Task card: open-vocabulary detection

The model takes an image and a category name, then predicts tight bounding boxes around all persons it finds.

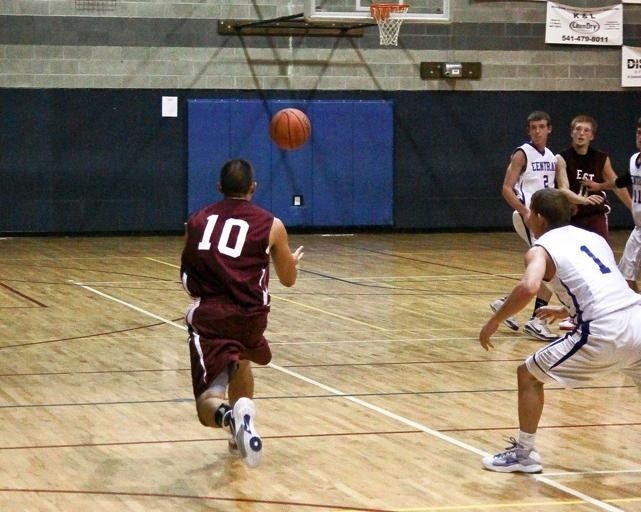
[479,188,641,474]
[487,110,560,341]
[555,115,631,329]
[178,160,305,469]
[619,120,641,291]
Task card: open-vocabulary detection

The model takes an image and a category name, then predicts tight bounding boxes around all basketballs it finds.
[269,107,310,151]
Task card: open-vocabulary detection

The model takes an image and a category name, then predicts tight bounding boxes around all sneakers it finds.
[228,435,243,457]
[488,294,522,332]
[558,314,577,330]
[231,396,264,466]
[481,438,544,474]
[522,317,562,343]
